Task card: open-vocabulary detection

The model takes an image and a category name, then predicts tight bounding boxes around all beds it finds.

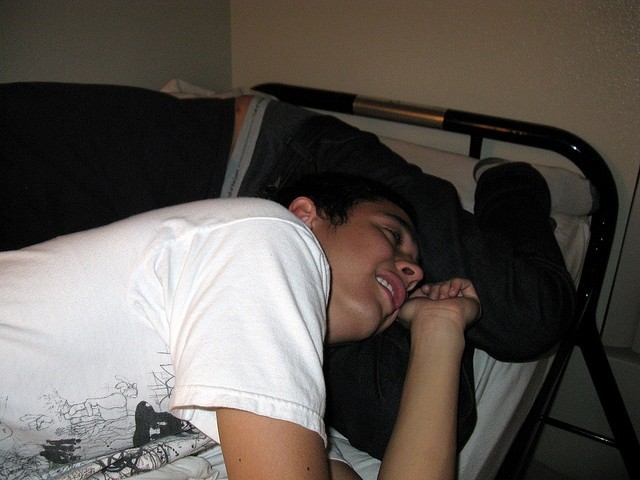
[0,81,640,478]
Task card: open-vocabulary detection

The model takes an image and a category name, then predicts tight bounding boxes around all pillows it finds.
[0,81,235,254]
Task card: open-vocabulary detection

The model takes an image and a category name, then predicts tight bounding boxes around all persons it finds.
[0,182,481,480]
